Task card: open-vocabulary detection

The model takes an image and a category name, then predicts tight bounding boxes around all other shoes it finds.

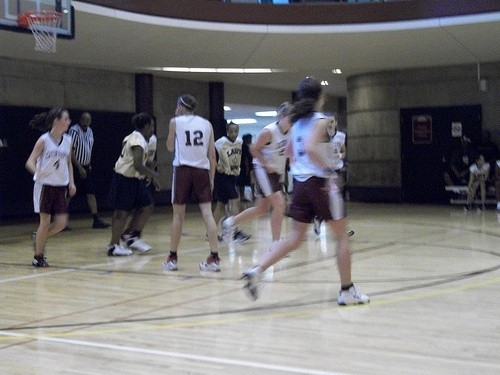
[93,222,110,229]
[61,226,72,232]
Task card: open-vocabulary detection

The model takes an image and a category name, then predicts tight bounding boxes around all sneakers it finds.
[219,216,240,247]
[161,256,177,270]
[199,255,220,271]
[338,287,370,305]
[31,232,37,251]
[120,233,152,252]
[32,254,49,267]
[347,231,354,237]
[314,216,321,234]
[241,272,259,300]
[106,243,132,256]
[234,231,252,244]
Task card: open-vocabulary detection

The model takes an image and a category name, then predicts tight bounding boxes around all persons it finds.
[465,154,490,212]
[494,160,500,210]
[25,106,76,267]
[218,100,294,256]
[107,112,158,256]
[313,112,356,239]
[240,134,257,203]
[242,77,368,306]
[206,121,250,243]
[281,157,290,192]
[164,94,221,272]
[61,111,109,231]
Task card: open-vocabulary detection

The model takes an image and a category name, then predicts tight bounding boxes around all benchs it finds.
[445,186,497,204]
